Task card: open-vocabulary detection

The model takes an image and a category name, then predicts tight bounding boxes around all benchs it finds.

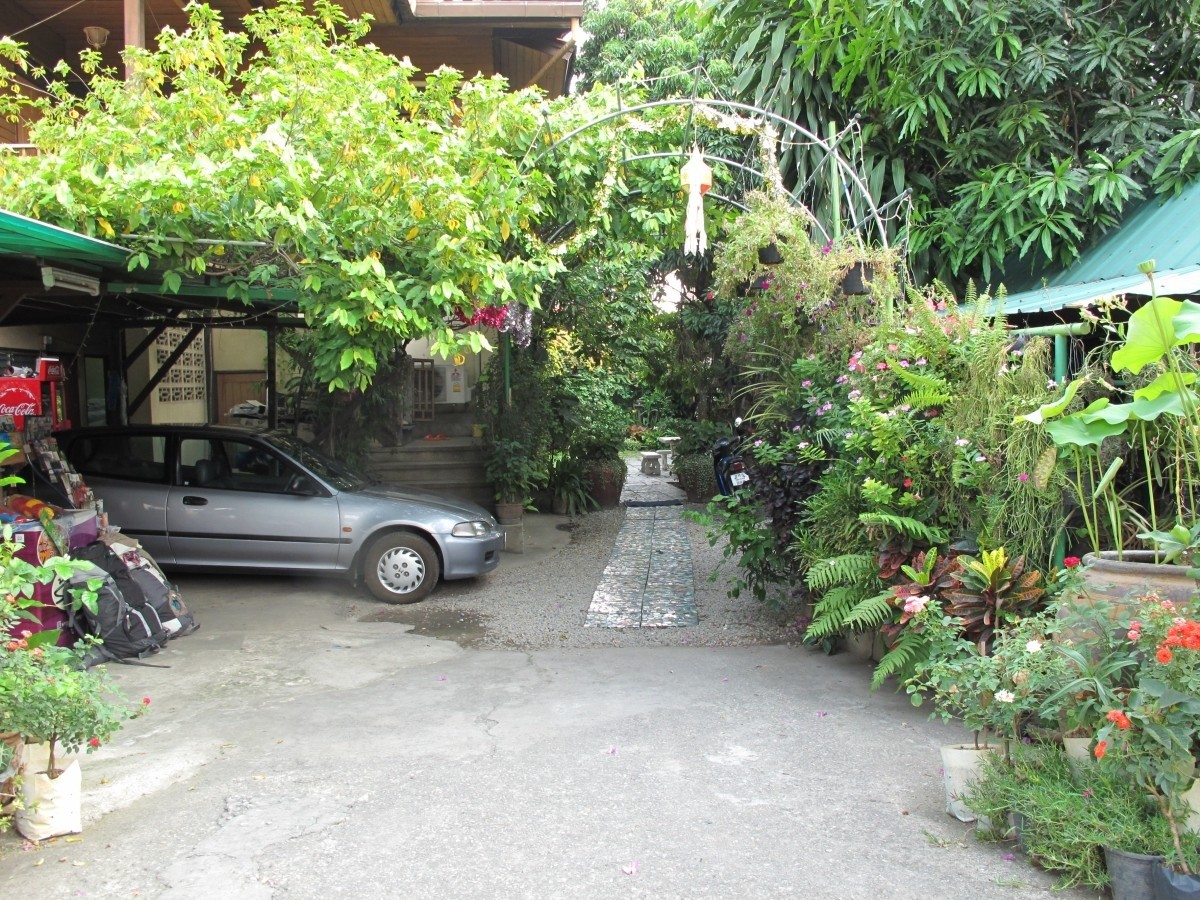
[657,450,671,470]
[641,451,662,476]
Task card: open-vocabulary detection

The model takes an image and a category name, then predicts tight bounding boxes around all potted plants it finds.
[485,439,539,523]
[548,459,604,516]
[0,441,102,802]
[901,255,1198,730]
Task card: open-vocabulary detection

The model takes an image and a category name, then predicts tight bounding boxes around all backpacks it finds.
[54,541,171,668]
[96,532,201,639]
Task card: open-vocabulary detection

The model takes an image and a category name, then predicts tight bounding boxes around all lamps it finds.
[41,266,100,297]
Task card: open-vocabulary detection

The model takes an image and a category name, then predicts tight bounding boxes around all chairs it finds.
[195,459,226,488]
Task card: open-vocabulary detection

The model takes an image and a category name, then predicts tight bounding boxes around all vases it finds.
[1152,857,1200,900]
[14,757,84,840]
[1098,842,1164,900]
[1062,732,1094,776]
[943,747,1004,822]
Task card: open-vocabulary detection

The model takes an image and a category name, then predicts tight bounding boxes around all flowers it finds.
[892,552,1198,884]
[0,639,151,779]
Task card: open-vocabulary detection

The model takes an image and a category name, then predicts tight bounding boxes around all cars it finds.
[2,424,507,605]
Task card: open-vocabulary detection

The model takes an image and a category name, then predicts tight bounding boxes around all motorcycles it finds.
[703,417,752,512]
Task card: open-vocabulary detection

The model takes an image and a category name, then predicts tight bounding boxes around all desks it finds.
[657,437,682,459]
